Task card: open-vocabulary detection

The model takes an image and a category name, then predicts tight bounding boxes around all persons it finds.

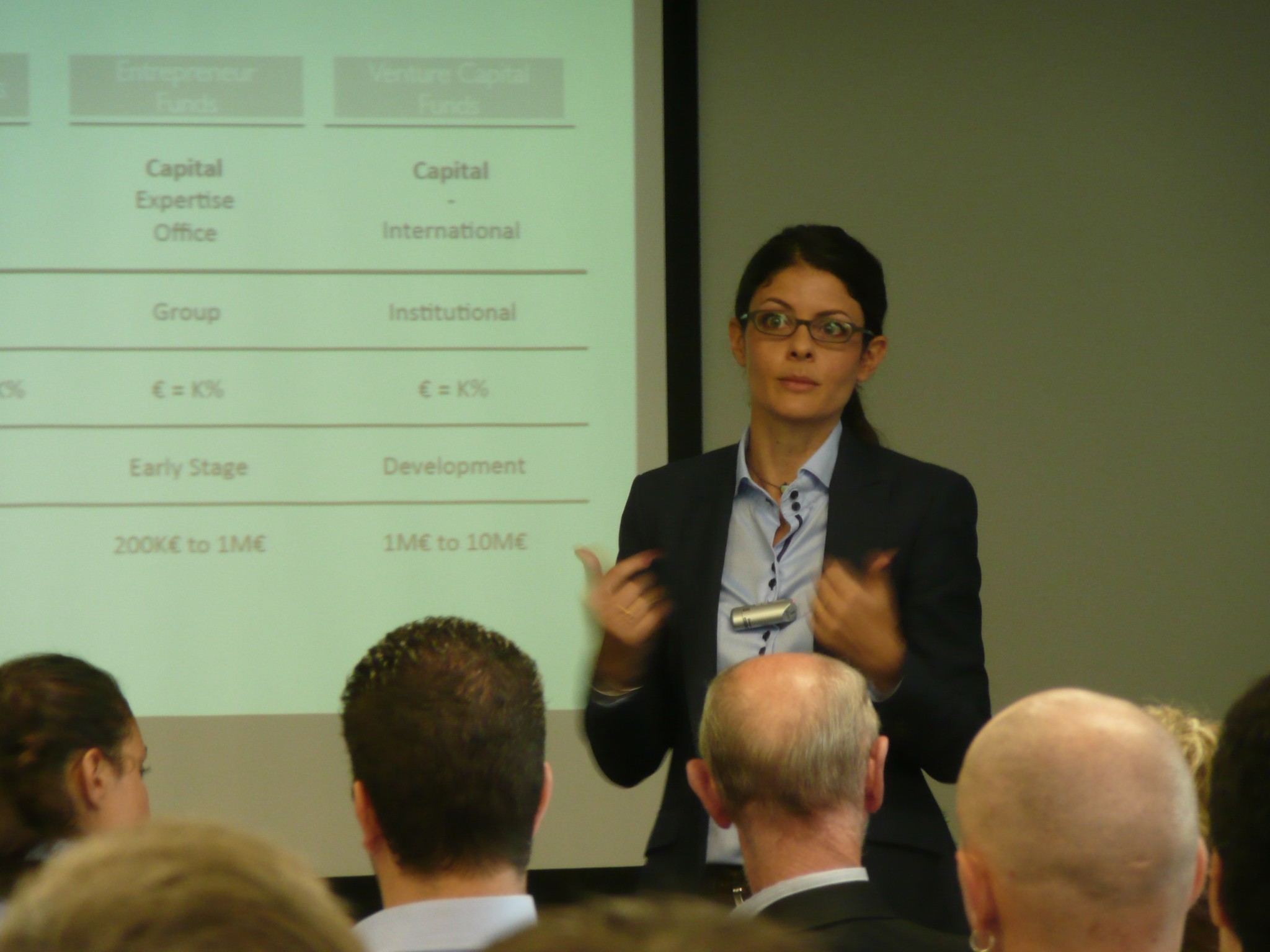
[0,615,1270,952]
[574,225,991,937]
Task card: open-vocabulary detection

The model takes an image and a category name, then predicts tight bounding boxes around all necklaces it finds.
[746,454,790,493]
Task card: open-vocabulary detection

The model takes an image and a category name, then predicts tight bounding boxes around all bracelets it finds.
[588,677,642,698]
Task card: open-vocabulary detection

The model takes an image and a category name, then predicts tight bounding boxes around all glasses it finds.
[738,309,872,342]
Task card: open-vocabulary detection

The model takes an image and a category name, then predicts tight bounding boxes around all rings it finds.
[623,607,641,620]
